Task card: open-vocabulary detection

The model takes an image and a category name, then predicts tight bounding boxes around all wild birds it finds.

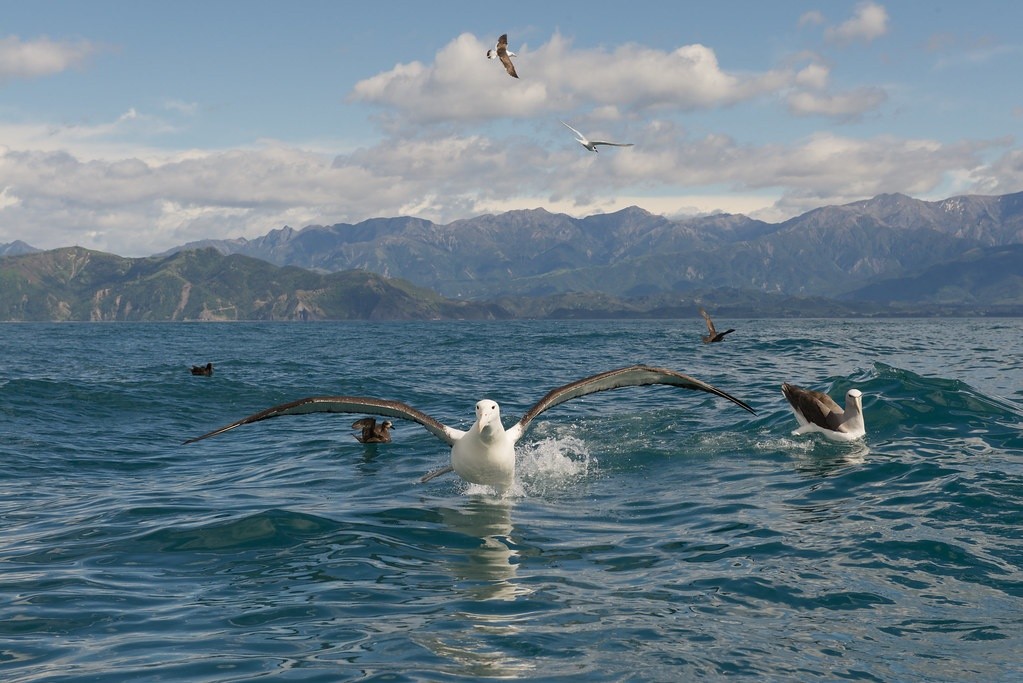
[487,33,519,79]
[351,416,396,445]
[180,362,758,498]
[701,309,735,343]
[559,120,634,155]
[781,380,867,443]
[189,363,214,377]
